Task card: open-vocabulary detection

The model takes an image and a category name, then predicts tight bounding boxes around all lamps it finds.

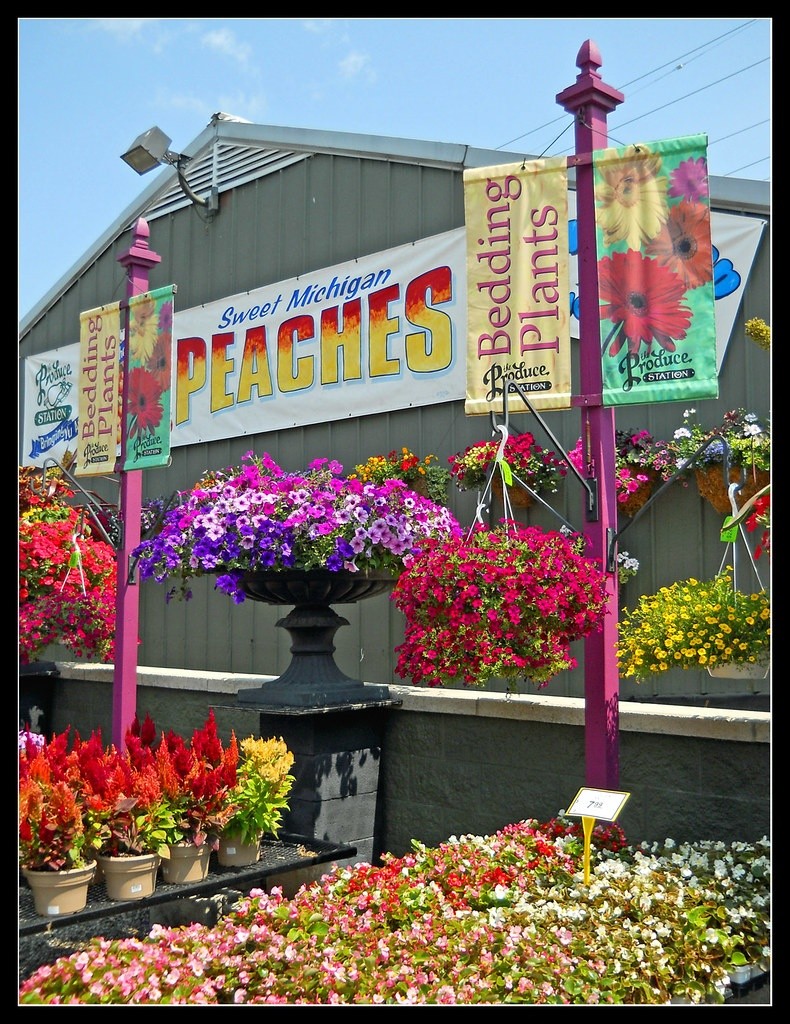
[120,125,173,176]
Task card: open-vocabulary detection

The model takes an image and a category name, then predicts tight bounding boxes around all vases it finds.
[217,828,264,867]
[695,464,770,515]
[400,476,429,500]
[489,468,535,508]
[160,840,213,885]
[22,859,97,917]
[707,652,770,681]
[97,852,162,902]
[727,963,770,998]
[616,463,663,518]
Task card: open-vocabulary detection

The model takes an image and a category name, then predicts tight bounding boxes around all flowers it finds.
[643,200,714,290]
[129,292,158,365]
[132,449,460,603]
[158,300,172,333]
[19,706,770,1005]
[146,331,171,392]
[560,525,640,585]
[594,144,670,252]
[448,433,568,494]
[613,566,770,684]
[653,408,770,488]
[745,496,770,561]
[346,446,454,505]
[19,488,142,664]
[127,366,164,439]
[667,156,710,202]
[564,428,656,502]
[389,516,613,690]
[598,248,694,358]
